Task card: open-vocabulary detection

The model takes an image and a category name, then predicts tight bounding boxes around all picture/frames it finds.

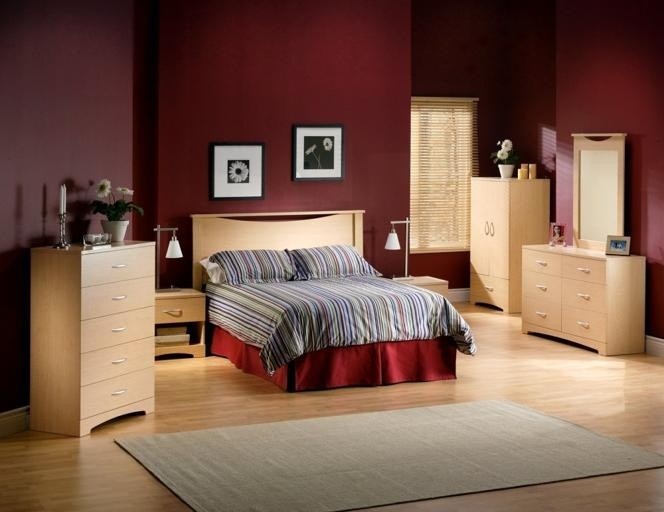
[291,121,347,183]
[604,235,632,257]
[206,139,266,202]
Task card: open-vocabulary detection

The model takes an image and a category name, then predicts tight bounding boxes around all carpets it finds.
[112,398,663,511]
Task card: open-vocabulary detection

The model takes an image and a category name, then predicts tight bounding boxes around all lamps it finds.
[384,216,415,280]
[154,222,185,293]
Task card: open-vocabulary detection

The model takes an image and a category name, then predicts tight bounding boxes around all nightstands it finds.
[399,276,449,298]
[155,287,207,359]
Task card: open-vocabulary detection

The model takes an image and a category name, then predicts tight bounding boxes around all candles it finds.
[64,185,66,213]
[60,185,64,216]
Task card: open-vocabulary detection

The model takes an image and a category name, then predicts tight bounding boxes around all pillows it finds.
[208,247,296,284]
[290,244,384,280]
[200,254,226,284]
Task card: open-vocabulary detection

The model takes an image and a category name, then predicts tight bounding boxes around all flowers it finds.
[90,178,145,219]
[303,137,333,169]
[226,160,249,184]
[495,139,513,163]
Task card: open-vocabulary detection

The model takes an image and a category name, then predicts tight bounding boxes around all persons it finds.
[553,226,561,238]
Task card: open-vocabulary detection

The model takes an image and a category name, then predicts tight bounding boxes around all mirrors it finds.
[570,131,627,252]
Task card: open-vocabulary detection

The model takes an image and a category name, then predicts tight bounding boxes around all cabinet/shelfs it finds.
[469,175,552,315]
[28,238,158,438]
[519,242,647,357]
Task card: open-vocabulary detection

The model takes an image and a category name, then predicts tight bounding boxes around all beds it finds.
[187,209,478,394]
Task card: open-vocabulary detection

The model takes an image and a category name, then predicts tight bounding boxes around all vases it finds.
[497,164,515,177]
[100,218,131,243]
